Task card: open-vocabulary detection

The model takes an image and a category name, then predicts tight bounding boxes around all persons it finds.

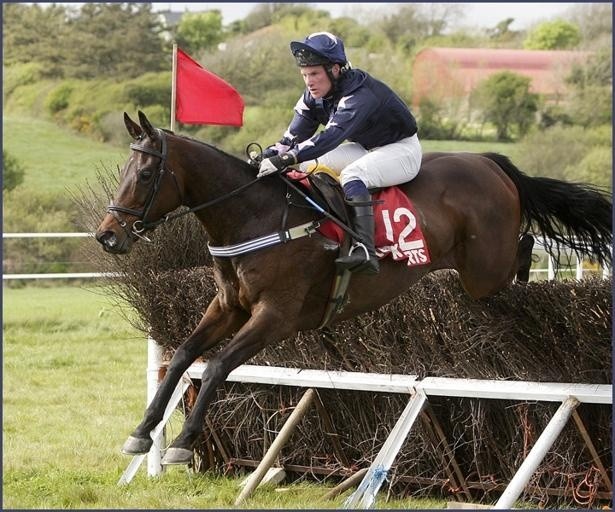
[247,32,424,277]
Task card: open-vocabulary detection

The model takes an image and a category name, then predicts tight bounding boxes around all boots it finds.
[331,193,379,276]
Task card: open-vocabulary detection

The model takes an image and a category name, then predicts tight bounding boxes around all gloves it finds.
[247,148,279,169]
[254,150,299,180]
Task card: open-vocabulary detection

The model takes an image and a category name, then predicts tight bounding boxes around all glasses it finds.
[294,49,334,67]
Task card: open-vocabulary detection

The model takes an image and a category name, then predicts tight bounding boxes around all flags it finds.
[174,44,245,128]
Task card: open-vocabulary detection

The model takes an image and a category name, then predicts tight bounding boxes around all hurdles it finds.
[70,156,614,510]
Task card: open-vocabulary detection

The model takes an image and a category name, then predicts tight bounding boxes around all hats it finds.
[288,31,347,64]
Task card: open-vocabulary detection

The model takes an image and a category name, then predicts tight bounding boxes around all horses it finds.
[94,108,614,467]
